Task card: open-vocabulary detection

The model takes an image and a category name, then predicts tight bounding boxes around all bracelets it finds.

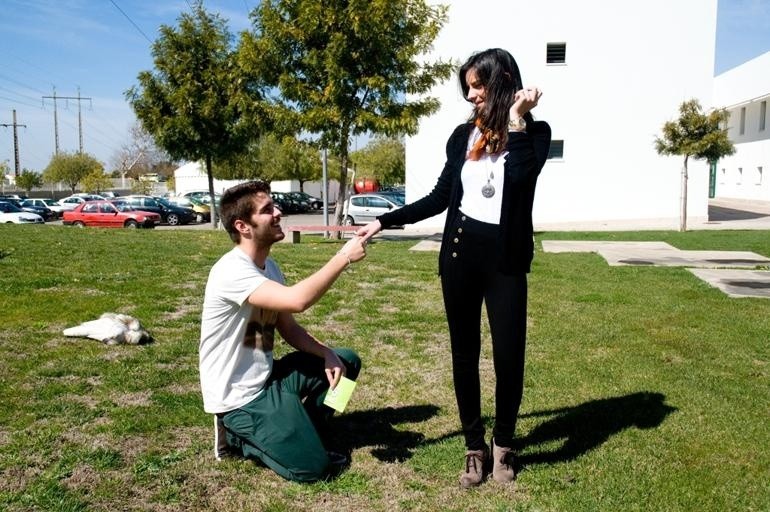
[336,250,351,270]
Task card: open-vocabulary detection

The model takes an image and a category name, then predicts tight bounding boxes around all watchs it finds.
[507,117,527,131]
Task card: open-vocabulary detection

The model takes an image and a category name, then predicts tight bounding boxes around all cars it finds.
[335,188,405,226]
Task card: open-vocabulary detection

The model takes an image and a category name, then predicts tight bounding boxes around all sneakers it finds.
[492,436,515,484]
[461,445,489,489]
[214,414,233,463]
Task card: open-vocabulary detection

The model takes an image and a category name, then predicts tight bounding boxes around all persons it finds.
[355,47,551,487]
[198,180,366,484]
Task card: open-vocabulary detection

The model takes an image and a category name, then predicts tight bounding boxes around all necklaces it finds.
[482,153,500,197]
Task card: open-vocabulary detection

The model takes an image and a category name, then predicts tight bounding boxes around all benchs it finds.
[286,225,373,244]
